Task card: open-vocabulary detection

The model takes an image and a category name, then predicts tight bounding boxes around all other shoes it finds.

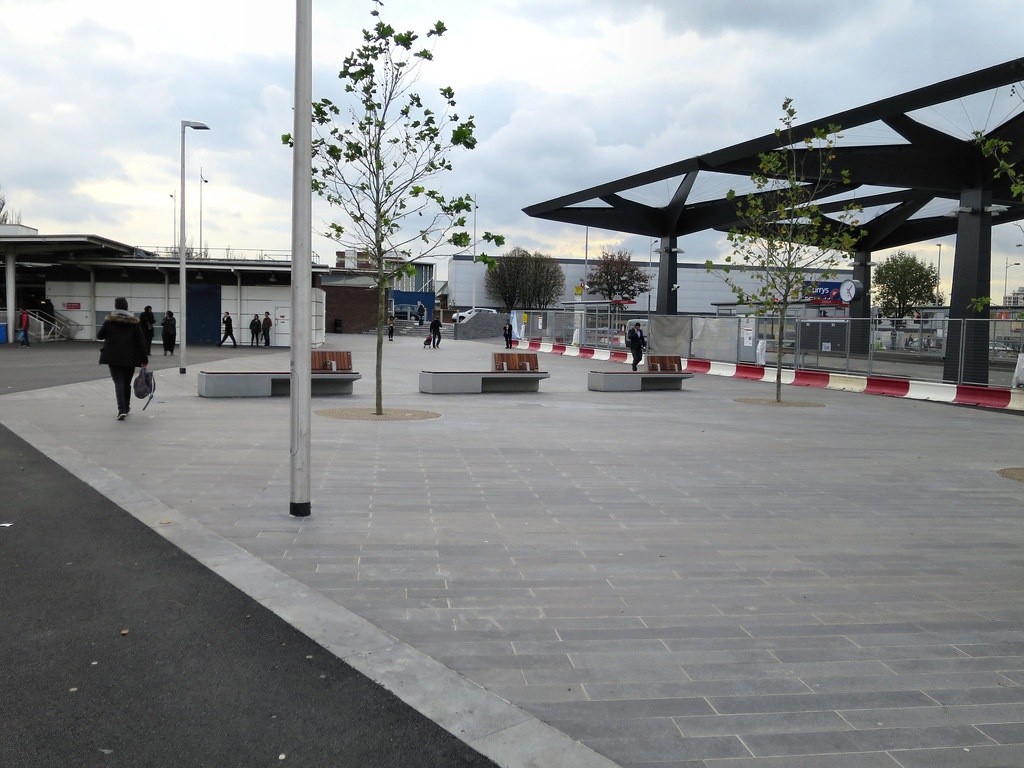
[231,346,237,348]
[216,342,220,347]
[164,353,173,355]
[389,338,393,341]
[433,345,439,348]
[263,346,270,349]
[20,345,30,349]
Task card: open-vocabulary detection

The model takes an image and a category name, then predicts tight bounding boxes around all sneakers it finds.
[117,407,130,420]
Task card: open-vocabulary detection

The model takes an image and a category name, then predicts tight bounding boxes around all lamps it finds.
[120,268,129,277]
[653,248,664,253]
[672,247,686,255]
[944,212,959,218]
[195,272,203,280]
[848,262,861,267]
[868,262,879,267]
[951,207,973,214]
[987,204,1012,216]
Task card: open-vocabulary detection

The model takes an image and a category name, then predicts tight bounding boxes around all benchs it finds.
[588,355,695,392]
[197,351,361,399]
[419,352,550,394]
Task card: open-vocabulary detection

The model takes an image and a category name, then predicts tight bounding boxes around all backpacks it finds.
[17,330,24,341]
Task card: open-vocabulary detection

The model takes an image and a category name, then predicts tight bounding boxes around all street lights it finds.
[179,120,210,373]
[1003,263,1020,306]
[472,205,479,309]
[937,243,942,306]
[648,240,659,311]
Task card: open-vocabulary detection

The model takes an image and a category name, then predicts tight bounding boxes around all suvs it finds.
[386,304,424,321]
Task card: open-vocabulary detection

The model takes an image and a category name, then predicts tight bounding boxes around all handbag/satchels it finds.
[133,365,155,399]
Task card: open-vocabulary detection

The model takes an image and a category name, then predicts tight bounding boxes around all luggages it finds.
[424,333,432,348]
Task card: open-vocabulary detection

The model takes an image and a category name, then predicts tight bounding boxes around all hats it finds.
[266,312,271,315]
[115,297,127,309]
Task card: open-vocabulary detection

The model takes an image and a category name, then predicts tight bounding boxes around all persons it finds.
[387,316,395,341]
[140,305,156,355]
[503,319,512,349]
[18,298,55,348]
[262,311,272,347]
[873,334,930,351]
[216,311,237,348]
[97,297,149,422]
[430,316,442,349]
[161,310,177,356]
[627,323,646,371]
[249,314,262,347]
[418,305,425,327]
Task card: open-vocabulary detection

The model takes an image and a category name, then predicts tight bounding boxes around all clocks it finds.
[840,280,855,301]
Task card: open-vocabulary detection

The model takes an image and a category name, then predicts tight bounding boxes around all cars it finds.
[452,308,497,323]
[989,342,1013,353]
